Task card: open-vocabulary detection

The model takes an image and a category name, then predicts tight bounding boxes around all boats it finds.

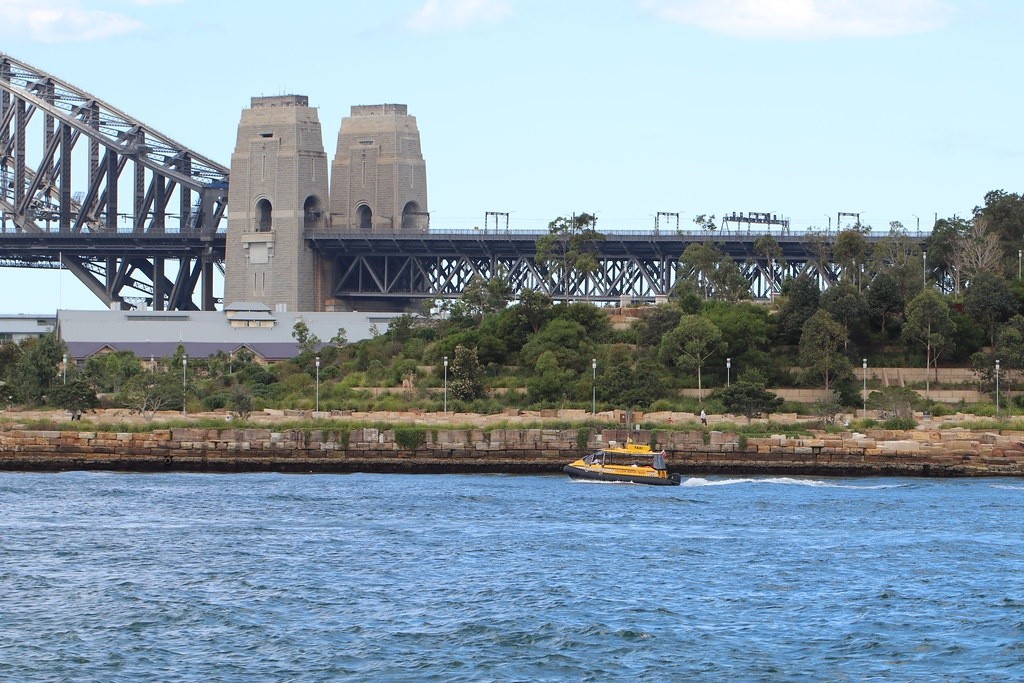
[563,437,681,487]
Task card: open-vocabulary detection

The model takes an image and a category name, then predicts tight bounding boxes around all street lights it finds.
[444,356,448,414]
[151,354,154,374]
[863,359,868,420]
[183,356,187,414]
[727,358,730,387]
[592,359,596,415]
[316,357,319,413]
[996,360,1000,413]
[63,354,67,385]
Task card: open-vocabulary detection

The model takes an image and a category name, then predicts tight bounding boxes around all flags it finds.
[662,451,666,458]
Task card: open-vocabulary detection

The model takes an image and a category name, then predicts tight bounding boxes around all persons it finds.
[700,408,706,425]
[593,457,600,463]
[630,462,637,467]
